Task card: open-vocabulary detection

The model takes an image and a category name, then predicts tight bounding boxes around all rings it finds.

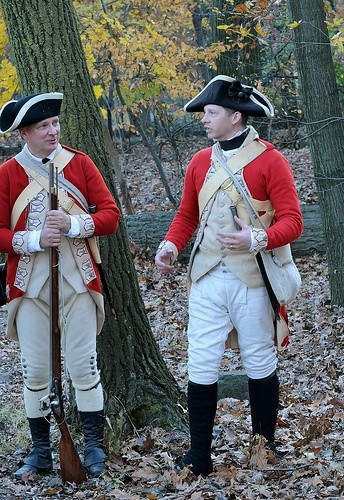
[231,245,233,248]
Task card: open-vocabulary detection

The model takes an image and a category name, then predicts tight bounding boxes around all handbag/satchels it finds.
[254,243,302,306]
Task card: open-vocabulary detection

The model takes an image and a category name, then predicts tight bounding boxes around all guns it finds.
[39,161,88,483]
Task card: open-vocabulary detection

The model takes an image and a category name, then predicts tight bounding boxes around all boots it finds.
[173,382,218,476]
[14,413,53,480]
[79,409,109,477]
[247,372,280,448]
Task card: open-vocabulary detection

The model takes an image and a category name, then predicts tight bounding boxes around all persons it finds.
[154,74,304,478]
[0,91,120,480]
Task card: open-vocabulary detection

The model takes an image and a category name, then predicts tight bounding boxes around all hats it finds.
[0,93,65,134]
[184,75,275,117]
